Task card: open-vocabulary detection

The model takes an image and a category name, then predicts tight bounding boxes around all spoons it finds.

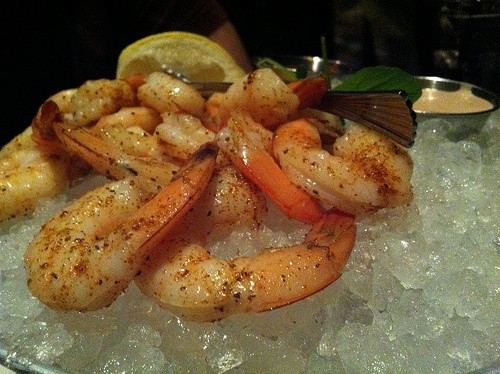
[159,67,418,150]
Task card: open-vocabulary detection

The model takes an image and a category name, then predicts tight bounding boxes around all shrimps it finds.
[2,69,415,325]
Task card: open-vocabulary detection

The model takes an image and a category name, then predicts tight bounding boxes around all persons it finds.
[0,0,252,151]
[336,0,405,69]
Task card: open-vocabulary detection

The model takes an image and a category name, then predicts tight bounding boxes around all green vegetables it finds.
[329,64,423,108]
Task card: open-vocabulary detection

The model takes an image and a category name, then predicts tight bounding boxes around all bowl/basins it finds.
[411,75,500,138]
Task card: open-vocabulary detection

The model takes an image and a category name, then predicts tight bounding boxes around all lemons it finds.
[116,30,244,87]
